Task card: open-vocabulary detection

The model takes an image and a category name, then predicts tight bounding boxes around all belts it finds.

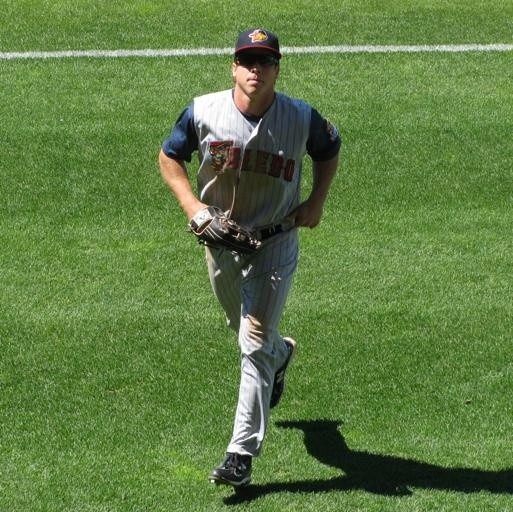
[252,220,293,240]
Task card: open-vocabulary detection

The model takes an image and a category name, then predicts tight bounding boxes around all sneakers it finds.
[269,337,297,409]
[207,452,252,486]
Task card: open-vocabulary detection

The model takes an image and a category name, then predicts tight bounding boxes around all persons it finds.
[158,28,341,486]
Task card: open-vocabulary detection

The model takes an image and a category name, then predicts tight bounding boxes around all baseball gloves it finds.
[189,205,262,255]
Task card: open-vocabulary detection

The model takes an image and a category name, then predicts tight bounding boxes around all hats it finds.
[235,28,281,59]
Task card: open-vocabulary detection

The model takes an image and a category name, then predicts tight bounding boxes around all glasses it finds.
[235,53,278,66]
